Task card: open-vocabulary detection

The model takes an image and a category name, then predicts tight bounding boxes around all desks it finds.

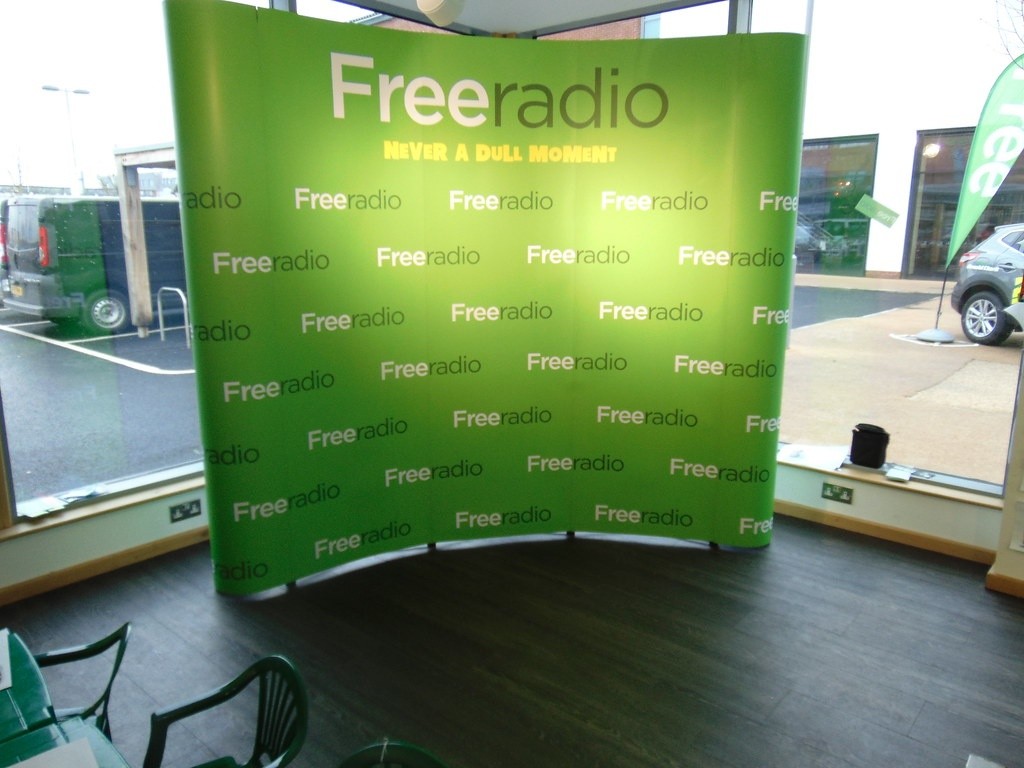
[0,632,132,768]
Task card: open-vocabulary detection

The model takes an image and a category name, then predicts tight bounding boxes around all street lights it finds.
[41,83,92,190]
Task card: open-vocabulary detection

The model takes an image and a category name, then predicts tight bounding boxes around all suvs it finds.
[948,222,1024,347]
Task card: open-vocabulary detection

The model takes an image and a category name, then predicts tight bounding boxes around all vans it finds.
[0,189,187,338]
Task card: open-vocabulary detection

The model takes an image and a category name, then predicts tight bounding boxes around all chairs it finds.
[336,740,450,768]
[34,620,133,743]
[142,654,309,768]
